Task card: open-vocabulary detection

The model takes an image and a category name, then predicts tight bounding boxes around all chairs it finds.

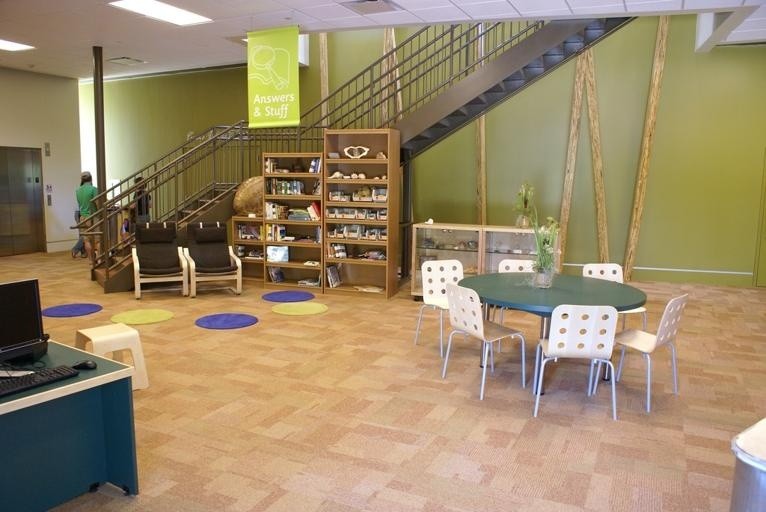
[582,263,647,333]
[183,221,243,298]
[605,293,688,414]
[532,304,619,420]
[442,283,526,400]
[492,259,536,353]
[132,222,188,299]
[415,259,464,357]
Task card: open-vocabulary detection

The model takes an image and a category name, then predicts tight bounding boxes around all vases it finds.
[515,215,530,229]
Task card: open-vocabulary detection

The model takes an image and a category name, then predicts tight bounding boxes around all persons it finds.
[125,176,152,235]
[76,171,108,266]
[71,181,89,261]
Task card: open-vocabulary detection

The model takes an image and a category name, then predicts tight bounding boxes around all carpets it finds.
[195,313,258,329]
[262,291,315,302]
[41,304,103,317]
[110,309,174,325]
[272,302,328,316]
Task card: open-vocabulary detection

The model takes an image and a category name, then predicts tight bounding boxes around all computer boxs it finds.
[0,342,48,366]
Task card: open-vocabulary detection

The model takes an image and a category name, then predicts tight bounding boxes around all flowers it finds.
[511,180,536,217]
[528,216,558,273]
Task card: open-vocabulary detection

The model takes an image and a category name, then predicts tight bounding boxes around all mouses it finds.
[73,359,97,370]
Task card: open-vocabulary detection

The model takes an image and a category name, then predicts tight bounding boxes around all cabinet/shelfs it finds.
[482,226,561,278]
[262,152,322,293]
[411,222,481,300]
[231,216,264,284]
[324,127,400,300]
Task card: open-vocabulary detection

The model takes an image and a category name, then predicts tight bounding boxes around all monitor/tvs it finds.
[0,279,48,353]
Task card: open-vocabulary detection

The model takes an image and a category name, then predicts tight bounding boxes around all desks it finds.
[454,272,646,396]
[0,341,141,512]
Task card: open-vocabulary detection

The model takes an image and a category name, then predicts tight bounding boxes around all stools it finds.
[76,322,149,392]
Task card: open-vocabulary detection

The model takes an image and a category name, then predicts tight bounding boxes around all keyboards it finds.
[0,365,79,396]
